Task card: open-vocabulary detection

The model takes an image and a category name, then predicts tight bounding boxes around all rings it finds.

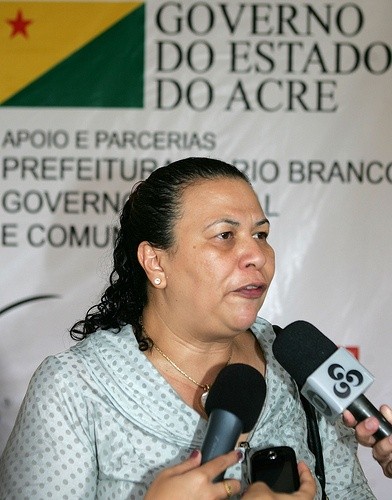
[224,480,233,497]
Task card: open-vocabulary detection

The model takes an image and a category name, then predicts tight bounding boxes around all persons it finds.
[0,156,377,500]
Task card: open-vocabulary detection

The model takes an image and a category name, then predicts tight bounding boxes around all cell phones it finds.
[250,445,300,494]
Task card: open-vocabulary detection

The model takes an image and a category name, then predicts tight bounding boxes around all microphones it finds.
[272,320,392,441]
[198,364,266,484]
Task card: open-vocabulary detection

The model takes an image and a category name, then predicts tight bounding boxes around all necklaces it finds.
[139,315,234,409]
